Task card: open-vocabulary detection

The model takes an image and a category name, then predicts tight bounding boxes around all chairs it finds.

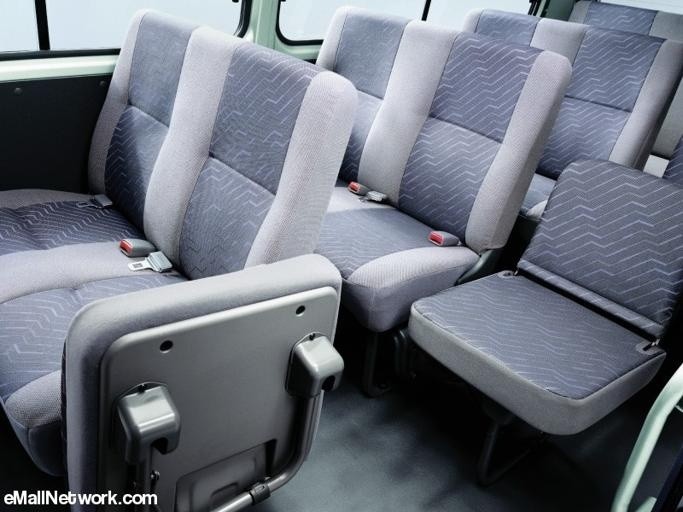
[0,0,682,511]
